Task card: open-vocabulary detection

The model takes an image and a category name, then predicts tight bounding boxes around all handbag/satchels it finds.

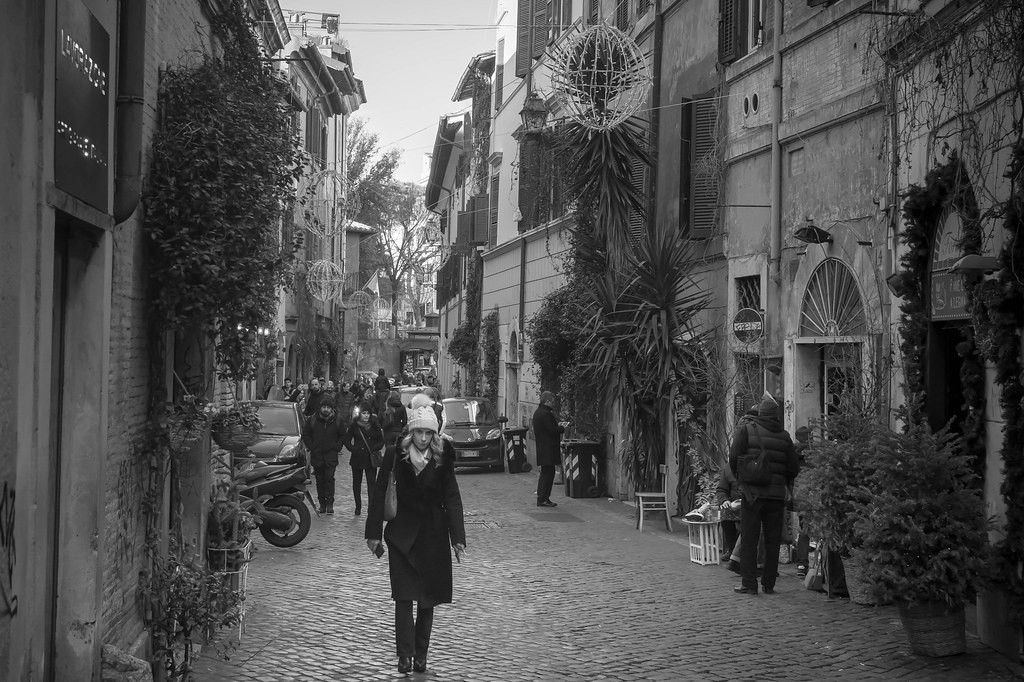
[370,451,383,467]
[384,451,398,520]
[737,423,768,484]
[381,402,394,428]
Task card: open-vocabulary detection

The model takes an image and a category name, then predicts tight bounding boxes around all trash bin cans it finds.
[565,440,603,498]
[560,439,588,496]
[502,425,532,474]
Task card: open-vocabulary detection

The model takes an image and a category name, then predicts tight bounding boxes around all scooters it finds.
[231,461,320,549]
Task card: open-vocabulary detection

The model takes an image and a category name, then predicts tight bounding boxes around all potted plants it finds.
[212,401,263,452]
[791,393,1005,657]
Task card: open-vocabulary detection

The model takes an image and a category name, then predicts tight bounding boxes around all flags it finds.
[368,275,379,293]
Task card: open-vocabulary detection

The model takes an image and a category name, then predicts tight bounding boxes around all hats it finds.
[409,394,438,433]
[360,401,373,414]
[760,391,779,415]
[320,394,333,410]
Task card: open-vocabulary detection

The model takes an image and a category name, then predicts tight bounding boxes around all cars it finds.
[439,397,509,474]
[395,387,442,413]
[234,399,314,476]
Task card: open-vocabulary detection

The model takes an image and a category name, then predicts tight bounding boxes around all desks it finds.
[682,518,724,565]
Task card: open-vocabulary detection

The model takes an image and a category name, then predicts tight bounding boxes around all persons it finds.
[365,394,466,672]
[718,397,814,595]
[265,368,437,514]
[533,391,569,507]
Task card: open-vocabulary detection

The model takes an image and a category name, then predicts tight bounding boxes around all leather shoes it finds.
[761,585,774,593]
[413,657,426,672]
[734,585,758,594]
[398,657,411,672]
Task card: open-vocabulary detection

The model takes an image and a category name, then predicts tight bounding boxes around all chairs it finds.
[634,464,673,533]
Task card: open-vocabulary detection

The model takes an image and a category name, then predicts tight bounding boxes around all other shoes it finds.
[355,507,361,515]
[797,565,807,575]
[537,499,557,507]
[319,498,326,513]
[327,499,334,514]
[726,560,741,574]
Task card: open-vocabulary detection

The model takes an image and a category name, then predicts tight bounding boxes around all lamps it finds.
[885,273,905,298]
[792,225,833,244]
[947,254,1001,275]
